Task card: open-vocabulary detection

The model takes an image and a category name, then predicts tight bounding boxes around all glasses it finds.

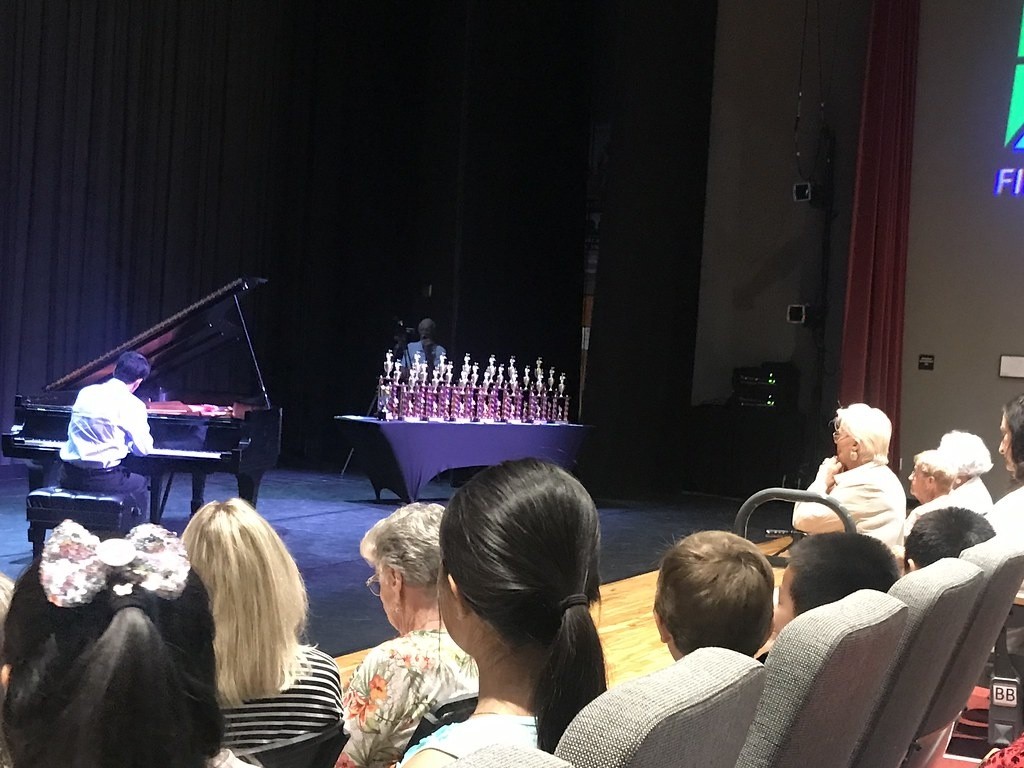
[833,432,848,444]
[366,570,382,596]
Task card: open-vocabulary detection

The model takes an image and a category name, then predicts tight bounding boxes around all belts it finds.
[69,464,118,473]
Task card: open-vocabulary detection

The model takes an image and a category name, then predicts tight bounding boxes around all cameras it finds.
[390,317,415,346]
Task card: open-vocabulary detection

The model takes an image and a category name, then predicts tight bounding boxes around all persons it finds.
[181,498,344,752]
[892,508,996,576]
[755,532,900,666]
[653,532,775,658]
[794,404,906,548]
[333,501,479,768]
[897,450,958,547]
[399,317,445,383]
[985,396,1024,655]
[59,352,154,534]
[0,519,258,768]
[398,461,607,768]
[938,429,993,514]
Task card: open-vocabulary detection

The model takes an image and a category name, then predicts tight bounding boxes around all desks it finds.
[335,415,598,506]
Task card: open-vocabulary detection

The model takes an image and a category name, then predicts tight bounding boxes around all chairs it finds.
[226,528,1024,768]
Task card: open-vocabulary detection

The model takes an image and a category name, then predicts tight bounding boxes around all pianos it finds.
[2,274,284,560]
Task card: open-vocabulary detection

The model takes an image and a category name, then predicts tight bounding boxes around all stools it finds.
[26,486,136,567]
